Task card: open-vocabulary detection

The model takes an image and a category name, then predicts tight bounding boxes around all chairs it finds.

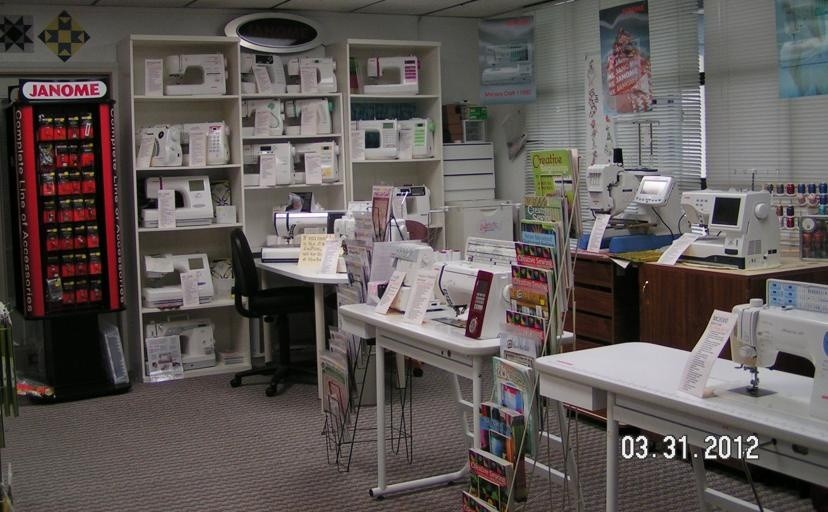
[230,228,334,399]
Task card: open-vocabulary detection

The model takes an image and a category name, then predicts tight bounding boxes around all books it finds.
[524,194,564,245]
[514,243,557,289]
[530,148,584,240]
[320,187,417,431]
[461,400,528,512]
[520,219,561,270]
[508,287,550,332]
[511,265,552,311]
[491,307,548,458]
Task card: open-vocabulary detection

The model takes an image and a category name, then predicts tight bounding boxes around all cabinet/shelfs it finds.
[563,248,640,350]
[328,38,449,251]
[443,138,497,206]
[239,88,346,192]
[114,32,248,383]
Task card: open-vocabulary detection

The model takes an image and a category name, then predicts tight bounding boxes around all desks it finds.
[256,252,408,416]
[628,248,827,359]
[338,303,577,499]
[531,339,827,512]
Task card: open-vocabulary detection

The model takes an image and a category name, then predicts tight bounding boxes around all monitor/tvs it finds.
[634,176,675,204]
[708,196,745,229]
